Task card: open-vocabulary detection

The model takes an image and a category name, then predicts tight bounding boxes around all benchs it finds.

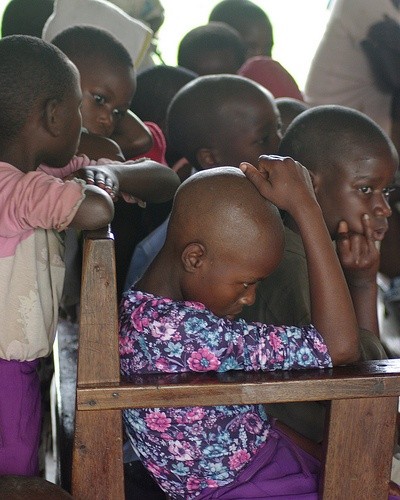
[69,227,400,500]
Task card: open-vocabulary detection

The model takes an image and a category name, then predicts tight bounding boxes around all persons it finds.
[119,155,362,500]
[0,0,163,500]
[0,35,182,500]
[130,1,400,488]
